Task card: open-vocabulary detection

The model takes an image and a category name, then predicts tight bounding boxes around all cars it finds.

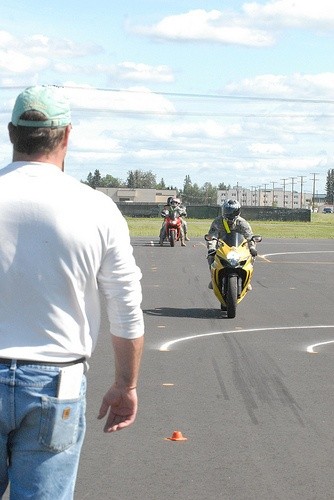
[323,208,332,213]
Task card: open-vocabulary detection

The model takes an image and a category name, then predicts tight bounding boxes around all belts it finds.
[0,357,85,365]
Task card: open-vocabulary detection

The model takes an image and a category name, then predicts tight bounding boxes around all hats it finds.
[12,85,71,127]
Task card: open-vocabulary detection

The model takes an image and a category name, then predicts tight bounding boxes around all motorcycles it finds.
[160,211,187,247]
[204,234,262,318]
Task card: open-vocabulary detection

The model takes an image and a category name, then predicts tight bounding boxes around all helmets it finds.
[222,198,241,224]
[167,197,173,206]
[172,199,180,207]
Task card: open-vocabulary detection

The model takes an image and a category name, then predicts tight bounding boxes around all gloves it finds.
[250,249,257,257]
[208,249,216,256]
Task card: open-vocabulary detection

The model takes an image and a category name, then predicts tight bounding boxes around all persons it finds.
[159,196,190,247]
[207,199,258,291]
[0,85,144,500]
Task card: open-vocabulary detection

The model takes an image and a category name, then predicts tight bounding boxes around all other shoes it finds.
[159,241,163,246]
[185,237,190,241]
[208,279,213,289]
[181,242,186,246]
[246,284,252,291]
